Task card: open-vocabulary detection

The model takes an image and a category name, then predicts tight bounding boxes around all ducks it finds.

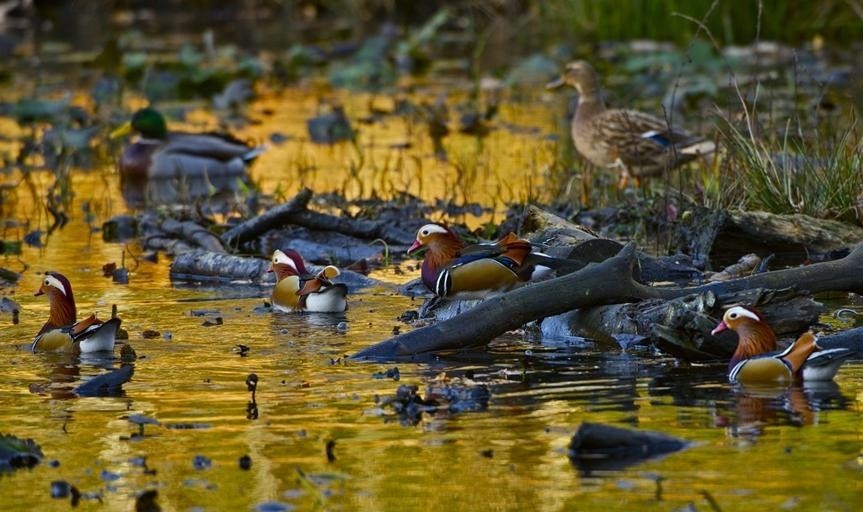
[109,107,268,175]
[544,58,723,200]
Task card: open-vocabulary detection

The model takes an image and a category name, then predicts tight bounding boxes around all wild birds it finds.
[407,222,551,296]
[710,303,856,383]
[31,273,121,352]
[266,248,348,313]
